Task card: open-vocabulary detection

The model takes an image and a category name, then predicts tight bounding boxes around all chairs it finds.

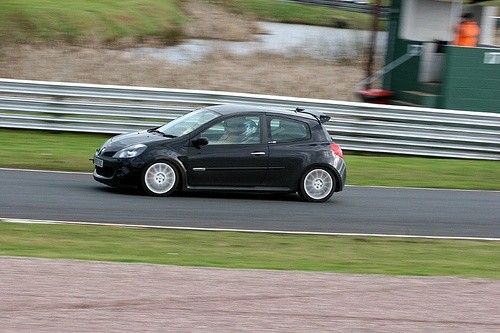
[271,120,306,142]
[215,114,247,144]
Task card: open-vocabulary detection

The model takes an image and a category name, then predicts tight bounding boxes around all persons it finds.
[452,13,480,47]
[216,116,244,144]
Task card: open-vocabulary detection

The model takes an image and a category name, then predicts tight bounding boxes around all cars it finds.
[89,105,347,202]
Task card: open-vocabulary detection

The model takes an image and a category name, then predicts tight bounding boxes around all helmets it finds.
[239,119,257,141]
